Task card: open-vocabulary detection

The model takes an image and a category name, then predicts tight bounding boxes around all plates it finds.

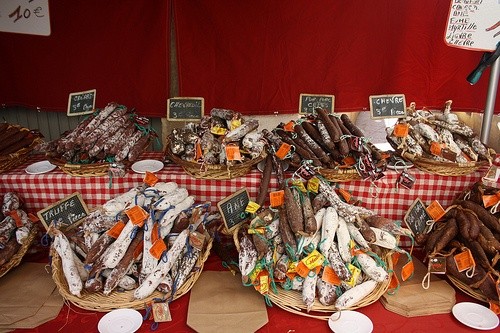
[97,308,144,333]
[257,158,289,174]
[452,301,499,330]
[387,162,415,170]
[130,160,164,173]
[25,160,56,174]
[328,310,374,333]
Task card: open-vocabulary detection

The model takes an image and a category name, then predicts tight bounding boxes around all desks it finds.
[0,144,499,251]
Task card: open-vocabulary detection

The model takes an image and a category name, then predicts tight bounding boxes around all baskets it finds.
[165,134,269,180]
[386,135,498,176]
[444,270,491,305]
[0,135,45,174]
[0,222,40,279]
[54,141,152,178]
[233,223,401,321]
[316,167,375,182]
[46,203,214,312]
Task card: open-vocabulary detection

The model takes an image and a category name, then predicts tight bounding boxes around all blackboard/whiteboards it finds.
[217,187,251,233]
[299,93,334,116]
[167,96,204,121]
[67,89,96,116]
[37,192,91,238]
[369,94,407,119]
[404,197,431,237]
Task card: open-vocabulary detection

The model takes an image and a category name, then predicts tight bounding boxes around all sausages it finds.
[384,99,498,165]
[0,181,212,302]
[239,175,408,312]
[411,178,500,305]
[0,100,154,162]
[169,106,381,168]
